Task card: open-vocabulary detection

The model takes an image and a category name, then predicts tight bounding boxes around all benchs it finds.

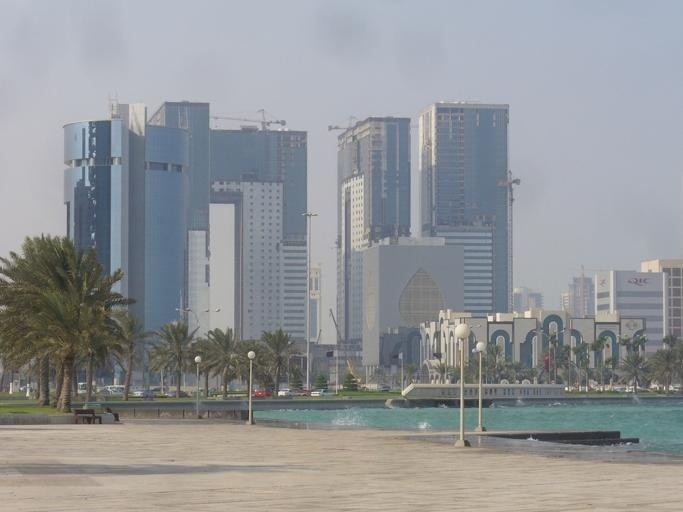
[74,408,102,424]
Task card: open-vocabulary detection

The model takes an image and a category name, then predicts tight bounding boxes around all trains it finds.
[401,383,568,399]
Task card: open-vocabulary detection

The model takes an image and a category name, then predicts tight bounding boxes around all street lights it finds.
[533,329,566,384]
[475,342,485,431]
[303,212,320,390]
[194,356,202,419]
[455,323,471,448]
[247,351,256,424]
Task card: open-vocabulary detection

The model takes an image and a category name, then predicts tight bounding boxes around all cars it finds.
[566,384,682,392]
[20,385,36,393]
[254,387,331,398]
[380,384,390,392]
[77,383,190,399]
